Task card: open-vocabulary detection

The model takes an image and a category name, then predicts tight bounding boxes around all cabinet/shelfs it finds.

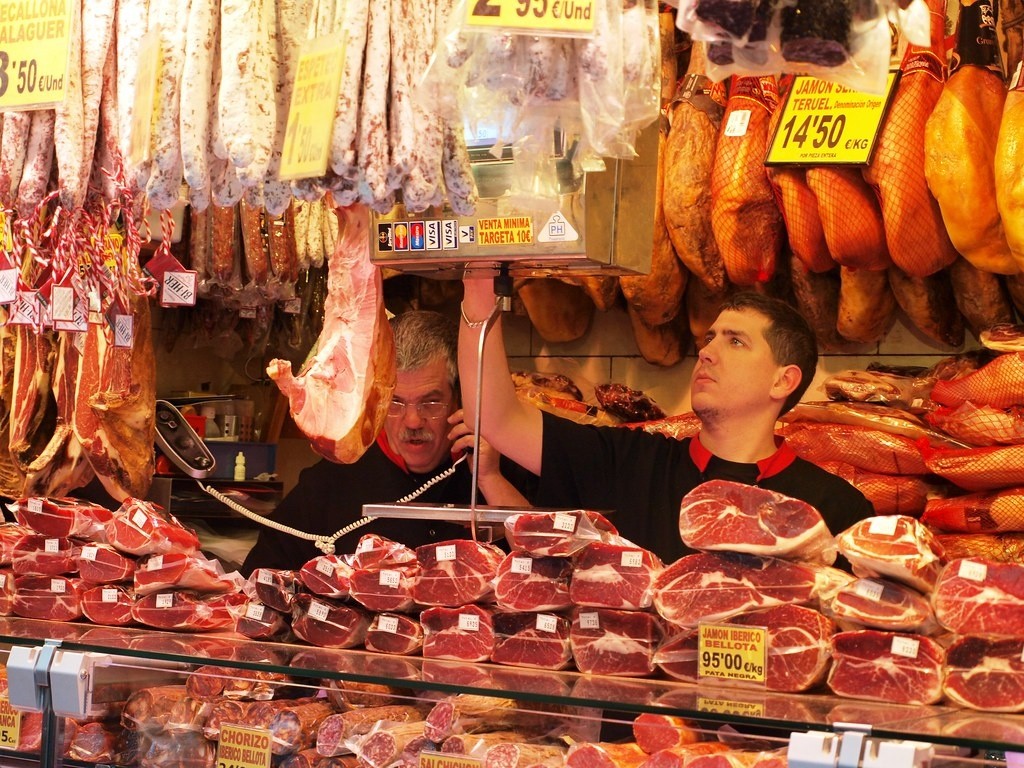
[369,119,659,276]
[1,613,1024,768]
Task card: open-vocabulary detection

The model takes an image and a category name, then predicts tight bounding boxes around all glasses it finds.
[386,395,453,420]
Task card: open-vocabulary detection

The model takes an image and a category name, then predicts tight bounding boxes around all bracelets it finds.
[460,303,484,328]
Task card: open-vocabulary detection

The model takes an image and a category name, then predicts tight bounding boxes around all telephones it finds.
[456,378,476,456]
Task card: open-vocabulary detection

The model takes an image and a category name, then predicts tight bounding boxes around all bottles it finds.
[235,452,246,481]
[202,407,220,439]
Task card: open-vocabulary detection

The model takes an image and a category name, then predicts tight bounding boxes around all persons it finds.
[457,262,875,743]
[239,310,571,700]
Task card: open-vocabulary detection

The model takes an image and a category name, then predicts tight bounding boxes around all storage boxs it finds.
[204,442,276,480]
